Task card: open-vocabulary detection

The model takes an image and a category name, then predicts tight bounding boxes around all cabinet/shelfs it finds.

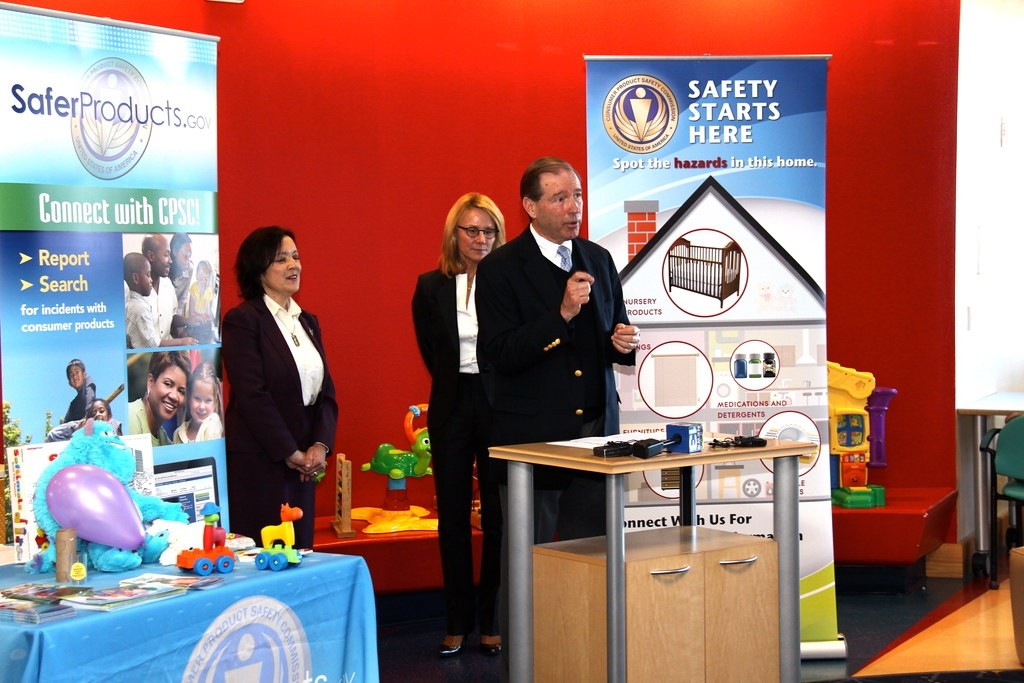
[487,438,818,681]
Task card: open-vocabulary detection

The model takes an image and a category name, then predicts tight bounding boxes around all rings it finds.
[313,472,317,476]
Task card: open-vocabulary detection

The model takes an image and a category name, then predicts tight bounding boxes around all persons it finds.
[129,351,224,449]
[221,226,338,552]
[411,192,506,660]
[45,359,123,443]
[122,233,219,350]
[474,156,641,657]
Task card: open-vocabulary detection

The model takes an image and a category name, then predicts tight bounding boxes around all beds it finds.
[668,237,741,308]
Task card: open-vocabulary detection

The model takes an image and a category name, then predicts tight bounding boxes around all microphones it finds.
[632,423,704,459]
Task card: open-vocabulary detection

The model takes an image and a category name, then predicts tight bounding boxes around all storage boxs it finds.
[1008,546,1024,665]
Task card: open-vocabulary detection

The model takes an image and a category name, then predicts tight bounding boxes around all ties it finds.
[557,244,572,273]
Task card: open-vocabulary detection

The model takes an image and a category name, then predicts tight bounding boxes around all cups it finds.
[56,528,77,583]
[70,553,88,585]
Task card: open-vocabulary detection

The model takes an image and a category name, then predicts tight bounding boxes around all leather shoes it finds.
[482,633,501,656]
[438,633,464,657]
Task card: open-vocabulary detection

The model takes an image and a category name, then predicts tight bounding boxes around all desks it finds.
[0,546,379,683]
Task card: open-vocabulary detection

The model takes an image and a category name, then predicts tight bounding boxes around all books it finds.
[0,573,226,625]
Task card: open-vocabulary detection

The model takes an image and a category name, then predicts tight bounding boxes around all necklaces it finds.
[467,285,472,291]
[276,313,300,346]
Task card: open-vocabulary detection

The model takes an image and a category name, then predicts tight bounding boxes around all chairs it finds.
[978,413,1024,591]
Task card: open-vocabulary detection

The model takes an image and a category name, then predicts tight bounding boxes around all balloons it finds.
[46,463,146,550]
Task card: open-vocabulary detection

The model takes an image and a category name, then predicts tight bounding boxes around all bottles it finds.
[734,354,747,378]
[748,353,763,378]
[763,352,776,378]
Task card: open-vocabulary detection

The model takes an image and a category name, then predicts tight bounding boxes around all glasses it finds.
[456,226,500,239]
